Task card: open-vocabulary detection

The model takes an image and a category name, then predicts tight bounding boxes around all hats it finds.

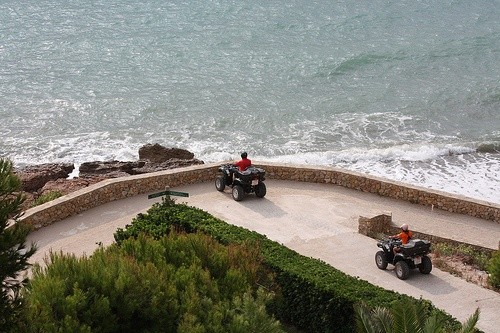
[241,152,247,157]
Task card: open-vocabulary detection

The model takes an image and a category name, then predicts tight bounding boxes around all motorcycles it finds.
[215,162,266,201]
[375,235,432,280]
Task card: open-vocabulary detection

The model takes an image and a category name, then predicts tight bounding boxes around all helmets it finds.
[400,224,408,230]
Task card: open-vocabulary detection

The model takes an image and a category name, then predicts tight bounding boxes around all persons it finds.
[389,223,414,255]
[229,152,252,187]
